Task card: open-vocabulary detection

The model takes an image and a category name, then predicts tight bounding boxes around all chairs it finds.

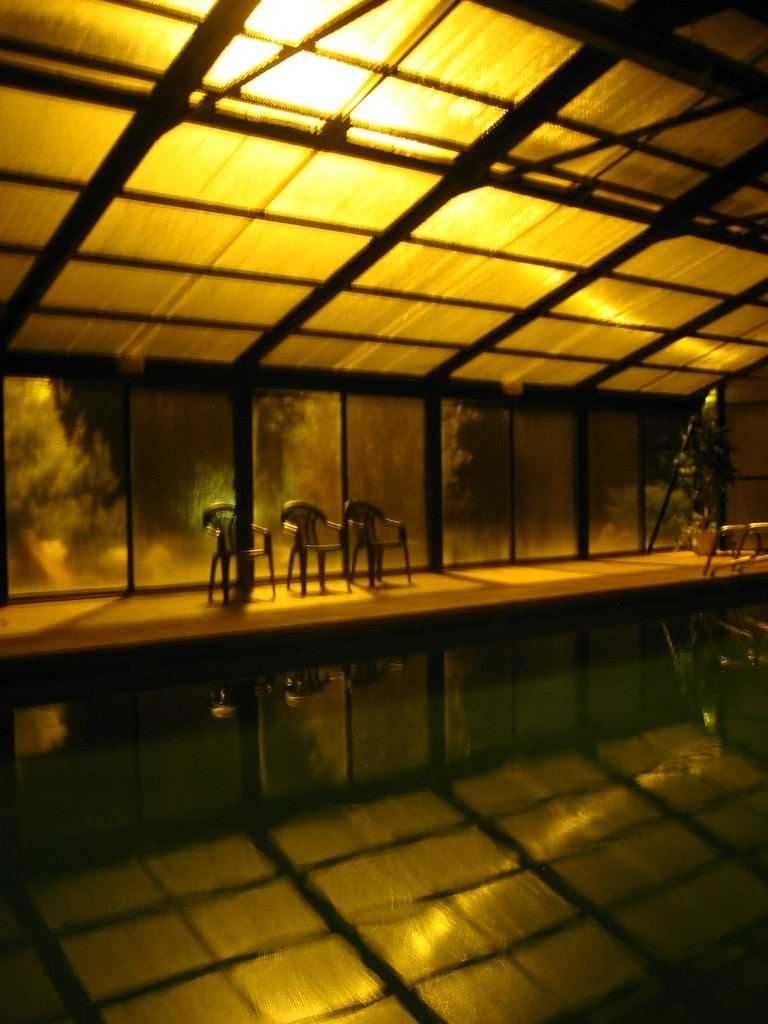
[207,652,413,720]
[203,502,277,606]
[343,498,413,589]
[279,498,354,598]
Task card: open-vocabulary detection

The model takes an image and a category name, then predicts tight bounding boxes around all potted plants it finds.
[678,414,738,556]
